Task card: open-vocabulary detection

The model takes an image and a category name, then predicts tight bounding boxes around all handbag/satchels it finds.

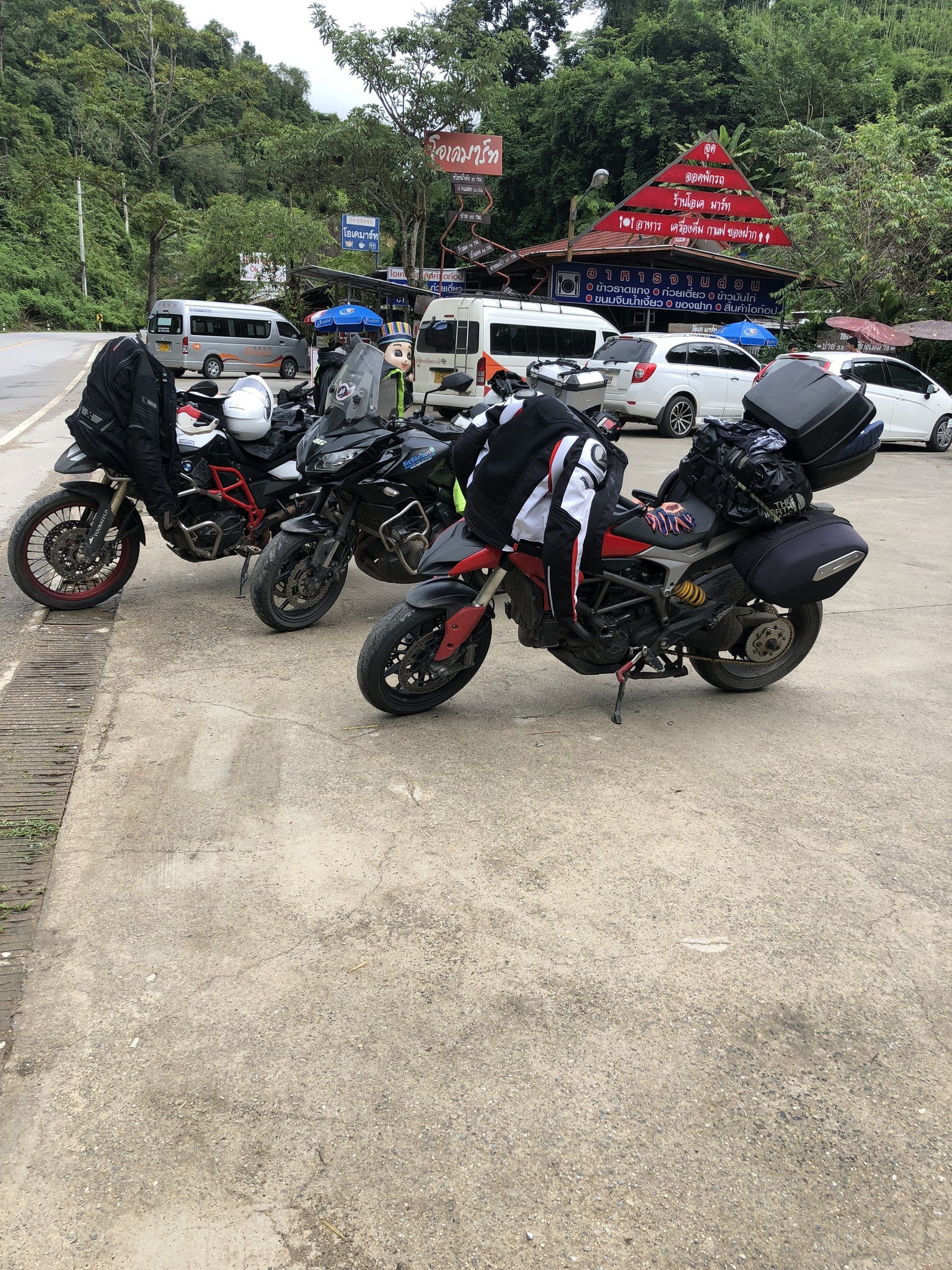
[678,415,813,533]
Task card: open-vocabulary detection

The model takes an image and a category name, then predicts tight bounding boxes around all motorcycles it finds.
[245,341,625,631]
[356,358,884,718]
[6,335,330,612]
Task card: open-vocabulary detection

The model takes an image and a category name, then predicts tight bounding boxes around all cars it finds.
[751,350,952,453]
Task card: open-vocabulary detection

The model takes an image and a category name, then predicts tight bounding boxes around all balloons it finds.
[315,305,382,341]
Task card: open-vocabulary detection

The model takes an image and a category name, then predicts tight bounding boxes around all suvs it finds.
[587,331,766,438]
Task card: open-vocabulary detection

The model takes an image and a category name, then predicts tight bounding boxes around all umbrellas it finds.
[892,318,952,376]
[709,319,778,347]
[304,310,328,324]
[825,315,914,353]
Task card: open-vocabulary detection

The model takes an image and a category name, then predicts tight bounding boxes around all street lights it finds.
[563,168,610,261]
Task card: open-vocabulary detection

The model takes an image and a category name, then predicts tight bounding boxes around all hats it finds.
[847,337,858,349]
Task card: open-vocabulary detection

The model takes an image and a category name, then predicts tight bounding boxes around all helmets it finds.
[175,404,227,454]
[221,374,274,441]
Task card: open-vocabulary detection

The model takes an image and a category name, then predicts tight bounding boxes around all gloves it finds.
[289,380,310,402]
[631,498,696,537]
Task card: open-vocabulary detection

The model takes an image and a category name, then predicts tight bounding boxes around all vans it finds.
[413,294,625,420]
[146,298,310,382]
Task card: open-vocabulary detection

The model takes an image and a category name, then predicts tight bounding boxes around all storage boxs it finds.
[740,356,890,492]
[743,510,870,609]
[527,354,609,422]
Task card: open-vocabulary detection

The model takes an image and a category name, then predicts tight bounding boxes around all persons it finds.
[845,337,862,353]
[788,343,799,360]
